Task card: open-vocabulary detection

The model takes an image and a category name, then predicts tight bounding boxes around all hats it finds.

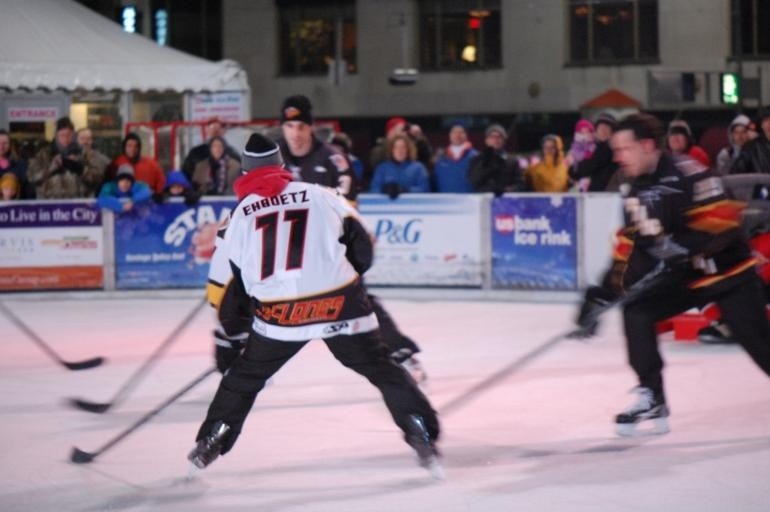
[56,116,74,131]
[593,113,614,129]
[117,163,135,183]
[281,95,312,125]
[667,118,691,141]
[241,132,286,173]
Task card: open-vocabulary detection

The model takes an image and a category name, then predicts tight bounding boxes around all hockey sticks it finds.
[1,303,103,370]
[71,367,218,464]
[64,296,207,414]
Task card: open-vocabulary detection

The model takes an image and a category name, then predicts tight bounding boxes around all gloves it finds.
[567,286,610,338]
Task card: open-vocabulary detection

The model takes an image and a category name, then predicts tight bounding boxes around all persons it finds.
[715,111,770,175]
[566,112,619,192]
[188,130,449,477]
[432,121,479,194]
[567,112,770,434]
[264,93,357,207]
[523,134,568,192]
[366,293,429,383]
[329,116,433,200]
[2,116,241,216]
[466,123,529,193]
[666,118,708,167]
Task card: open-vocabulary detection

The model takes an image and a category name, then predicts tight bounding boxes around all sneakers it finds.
[699,318,737,344]
[389,335,417,362]
[410,437,438,465]
[617,387,669,421]
[191,437,220,466]
